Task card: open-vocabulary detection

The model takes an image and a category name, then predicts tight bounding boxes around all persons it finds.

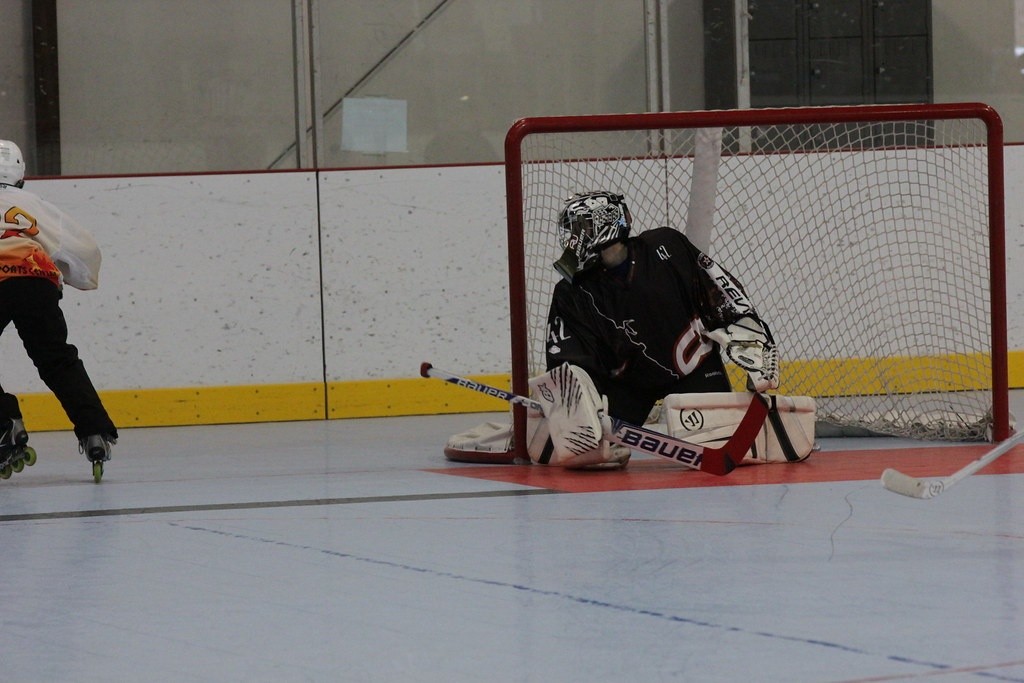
[0,139,119,462]
[545,191,778,448]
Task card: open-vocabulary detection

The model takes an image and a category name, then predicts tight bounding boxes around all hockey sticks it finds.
[879,429,1024,499]
[419,361,771,474]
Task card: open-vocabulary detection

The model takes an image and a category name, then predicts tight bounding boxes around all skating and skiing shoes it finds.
[0,393,37,480]
[74,417,118,483]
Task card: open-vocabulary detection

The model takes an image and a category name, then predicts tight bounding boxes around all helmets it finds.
[0,140,25,186]
[558,191,631,273]
